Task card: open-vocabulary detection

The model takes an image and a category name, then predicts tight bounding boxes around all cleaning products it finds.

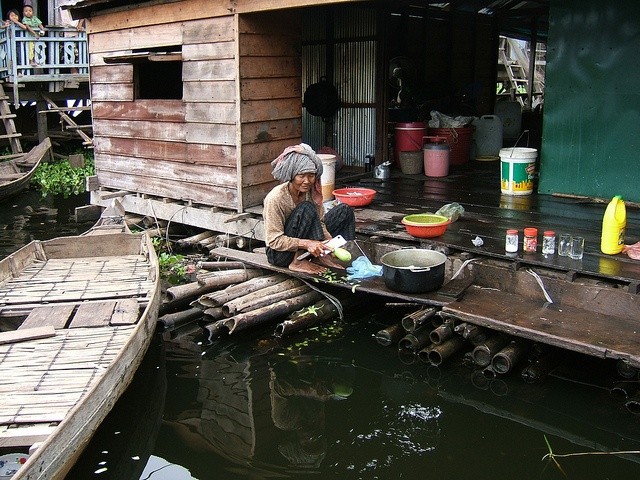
[600,194,627,254]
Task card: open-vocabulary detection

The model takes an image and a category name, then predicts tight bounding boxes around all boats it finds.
[1,134,52,195]
[0,197,162,480]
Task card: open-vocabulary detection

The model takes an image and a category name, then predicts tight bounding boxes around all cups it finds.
[557,233,571,256]
[570,237,584,260]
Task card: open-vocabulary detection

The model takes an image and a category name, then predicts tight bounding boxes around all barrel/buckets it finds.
[499,129,538,196]
[314,153,337,202]
[394,122,425,152]
[421,136,450,177]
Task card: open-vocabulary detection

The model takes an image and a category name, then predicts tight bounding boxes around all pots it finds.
[381,246,447,293]
[305,76,338,123]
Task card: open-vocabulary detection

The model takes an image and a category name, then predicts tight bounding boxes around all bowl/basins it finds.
[333,188,377,207]
[474,155,499,168]
[401,214,450,238]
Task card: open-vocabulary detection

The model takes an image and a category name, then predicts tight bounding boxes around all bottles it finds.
[542,231,555,254]
[505,230,518,252]
[523,228,538,251]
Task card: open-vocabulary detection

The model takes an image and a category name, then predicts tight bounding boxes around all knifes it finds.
[295,235,348,260]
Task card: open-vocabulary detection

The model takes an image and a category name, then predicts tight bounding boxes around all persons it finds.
[263,142,356,267]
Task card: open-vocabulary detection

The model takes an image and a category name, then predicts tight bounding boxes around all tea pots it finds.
[373,160,393,178]
